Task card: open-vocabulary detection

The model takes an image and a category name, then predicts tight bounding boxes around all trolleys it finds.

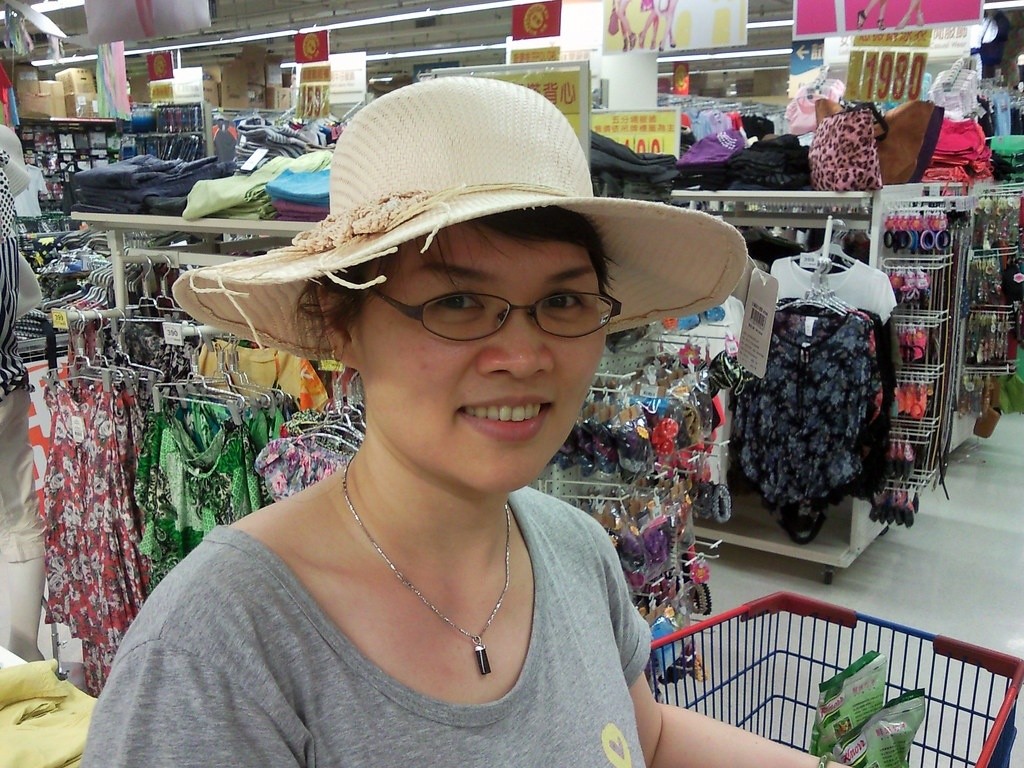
[652,591,1024,767]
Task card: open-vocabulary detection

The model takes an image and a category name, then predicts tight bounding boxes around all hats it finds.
[171,76,747,360]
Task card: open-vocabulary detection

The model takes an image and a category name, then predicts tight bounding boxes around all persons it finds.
[79,76,861,768]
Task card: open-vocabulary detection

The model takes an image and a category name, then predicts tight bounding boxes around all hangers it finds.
[723,198,871,319]
[209,105,362,130]
[925,58,1024,116]
[40,231,367,455]
[53,154,82,176]
[657,66,838,121]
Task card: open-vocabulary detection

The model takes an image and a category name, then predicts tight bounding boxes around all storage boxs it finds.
[148,44,291,109]
[4,64,99,118]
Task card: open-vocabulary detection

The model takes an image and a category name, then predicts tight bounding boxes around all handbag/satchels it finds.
[878,100,944,184]
[808,102,889,190]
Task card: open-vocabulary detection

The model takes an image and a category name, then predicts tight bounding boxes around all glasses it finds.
[359,284,622,342]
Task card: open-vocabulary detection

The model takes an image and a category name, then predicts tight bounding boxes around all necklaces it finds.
[344,446,511,674]
